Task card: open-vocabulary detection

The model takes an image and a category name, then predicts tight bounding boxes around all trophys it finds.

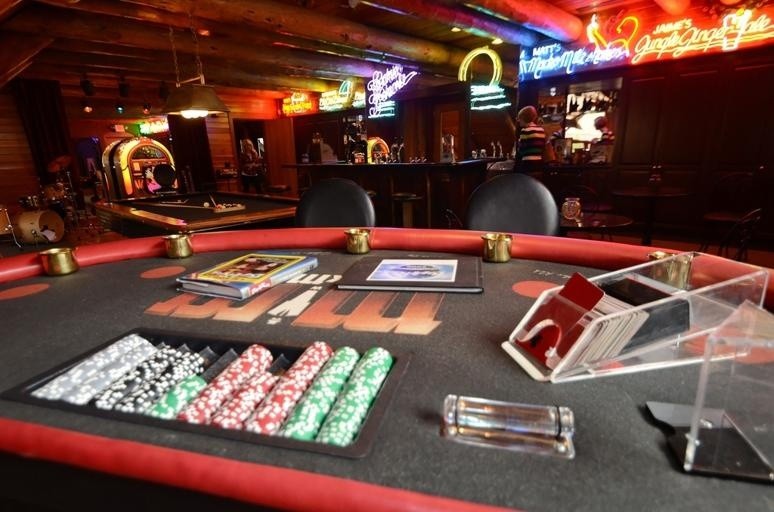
[209,194,216,206]
[130,202,213,210]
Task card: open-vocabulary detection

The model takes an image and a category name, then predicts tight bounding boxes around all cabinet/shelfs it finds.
[472,141,503,159]
[391,136,406,163]
[569,91,618,112]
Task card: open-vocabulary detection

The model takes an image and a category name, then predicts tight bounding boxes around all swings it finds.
[440,133,455,164]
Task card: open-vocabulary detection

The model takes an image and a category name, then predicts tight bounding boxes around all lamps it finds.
[294,171,558,243]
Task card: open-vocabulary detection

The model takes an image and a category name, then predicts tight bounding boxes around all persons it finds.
[589,116,616,146]
[511,107,556,161]
[545,118,564,146]
[238,139,263,194]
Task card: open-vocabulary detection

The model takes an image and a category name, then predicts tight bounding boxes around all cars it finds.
[343,229,371,254]
[647,252,694,290]
[162,233,193,258]
[481,232,512,263]
[39,247,78,276]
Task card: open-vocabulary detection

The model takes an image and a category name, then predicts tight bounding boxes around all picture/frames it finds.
[93,191,299,234]
